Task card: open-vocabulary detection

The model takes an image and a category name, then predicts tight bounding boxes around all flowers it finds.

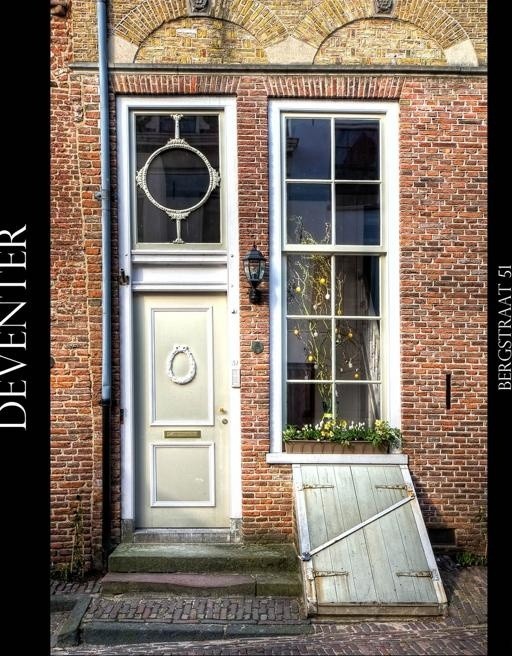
[284,413,400,450]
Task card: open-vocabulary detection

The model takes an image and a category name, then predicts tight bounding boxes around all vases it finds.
[284,437,390,454]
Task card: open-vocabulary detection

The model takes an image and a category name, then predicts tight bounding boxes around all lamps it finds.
[243,240,266,303]
